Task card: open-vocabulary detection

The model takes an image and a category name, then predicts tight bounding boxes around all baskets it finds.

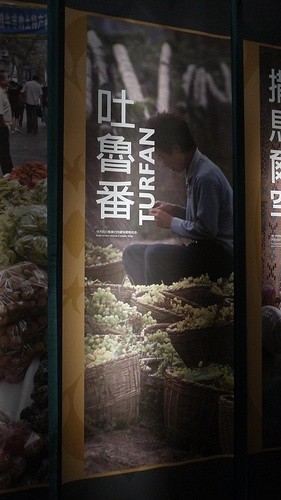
[172,286,234,315]
[135,291,201,330]
[147,368,167,428]
[173,312,234,371]
[87,253,130,284]
[82,336,144,429]
[140,356,163,378]
[219,390,238,448]
[163,362,232,437]
[84,277,131,309]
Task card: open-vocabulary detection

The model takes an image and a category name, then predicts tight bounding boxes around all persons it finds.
[0,67,49,175]
[123,110,234,289]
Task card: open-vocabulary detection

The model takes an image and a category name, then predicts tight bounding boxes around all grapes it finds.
[85,240,235,392]
